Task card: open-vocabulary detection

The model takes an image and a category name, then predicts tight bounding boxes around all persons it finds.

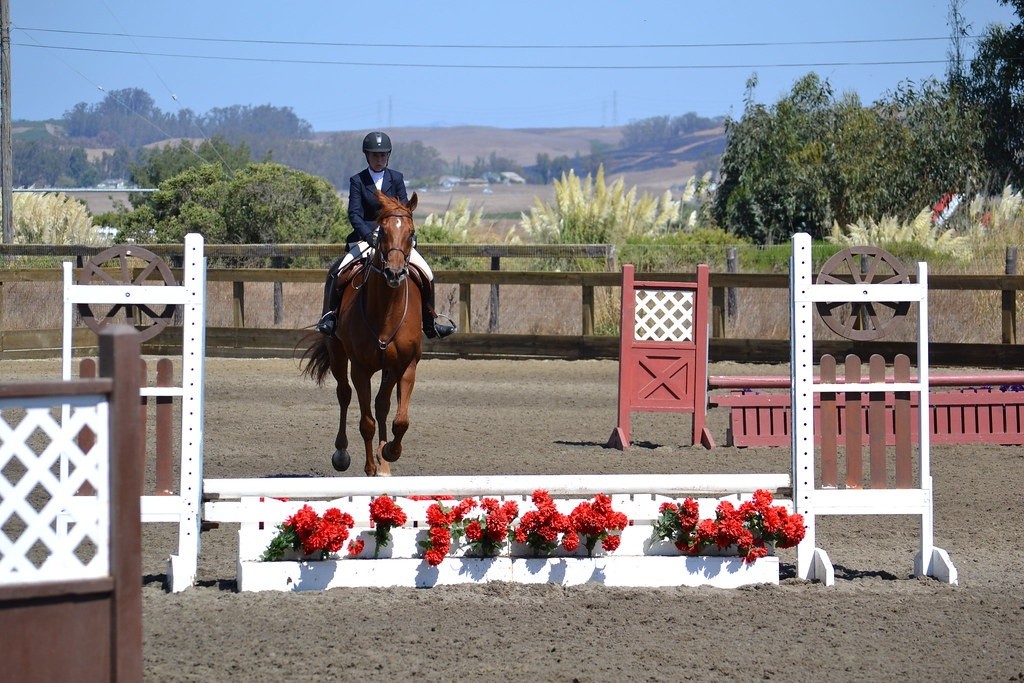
[316,131,455,339]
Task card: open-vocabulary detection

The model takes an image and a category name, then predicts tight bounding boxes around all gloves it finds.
[411,235,417,248]
[366,233,378,248]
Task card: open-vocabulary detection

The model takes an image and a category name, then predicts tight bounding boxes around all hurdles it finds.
[61,232,959,587]
[604,263,1024,452]
[0,322,145,683]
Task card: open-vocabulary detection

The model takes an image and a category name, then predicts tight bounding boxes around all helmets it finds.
[363,131,392,153]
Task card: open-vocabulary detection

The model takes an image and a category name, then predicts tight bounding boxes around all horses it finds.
[292,189,424,476]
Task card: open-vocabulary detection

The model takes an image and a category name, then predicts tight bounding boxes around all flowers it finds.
[262,489,630,565]
[651,491,805,562]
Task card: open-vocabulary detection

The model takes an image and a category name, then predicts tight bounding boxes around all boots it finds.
[317,275,338,335]
[419,279,455,339]
